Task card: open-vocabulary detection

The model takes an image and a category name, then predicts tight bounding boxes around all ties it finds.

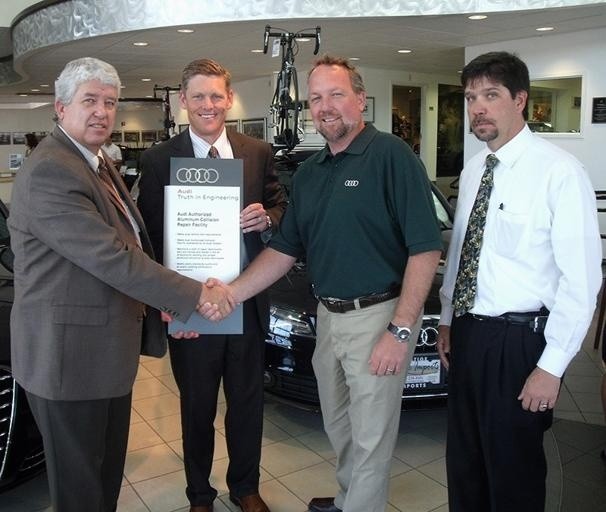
[451,154,499,318]
[97,156,120,198]
[209,145,218,159]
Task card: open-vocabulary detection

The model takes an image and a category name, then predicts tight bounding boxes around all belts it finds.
[474,313,535,327]
[318,290,400,314]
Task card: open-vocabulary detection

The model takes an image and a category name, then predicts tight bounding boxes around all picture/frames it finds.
[364,97,375,123]
[179,118,267,144]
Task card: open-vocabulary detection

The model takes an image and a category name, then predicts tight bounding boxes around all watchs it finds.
[386,321,412,343]
[259,213,273,234]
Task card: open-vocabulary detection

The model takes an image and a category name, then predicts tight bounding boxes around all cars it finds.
[117,145,149,188]
[0,200,44,494]
[260,150,458,416]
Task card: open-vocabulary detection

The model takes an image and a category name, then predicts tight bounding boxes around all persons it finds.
[434,48,602,511]
[6,56,236,512]
[137,58,288,512]
[194,57,445,512]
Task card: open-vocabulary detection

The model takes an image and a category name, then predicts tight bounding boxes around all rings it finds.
[540,404,548,408]
[386,368,394,373]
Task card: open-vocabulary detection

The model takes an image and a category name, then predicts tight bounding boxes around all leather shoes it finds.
[229,490,270,512]
[308,497,343,512]
